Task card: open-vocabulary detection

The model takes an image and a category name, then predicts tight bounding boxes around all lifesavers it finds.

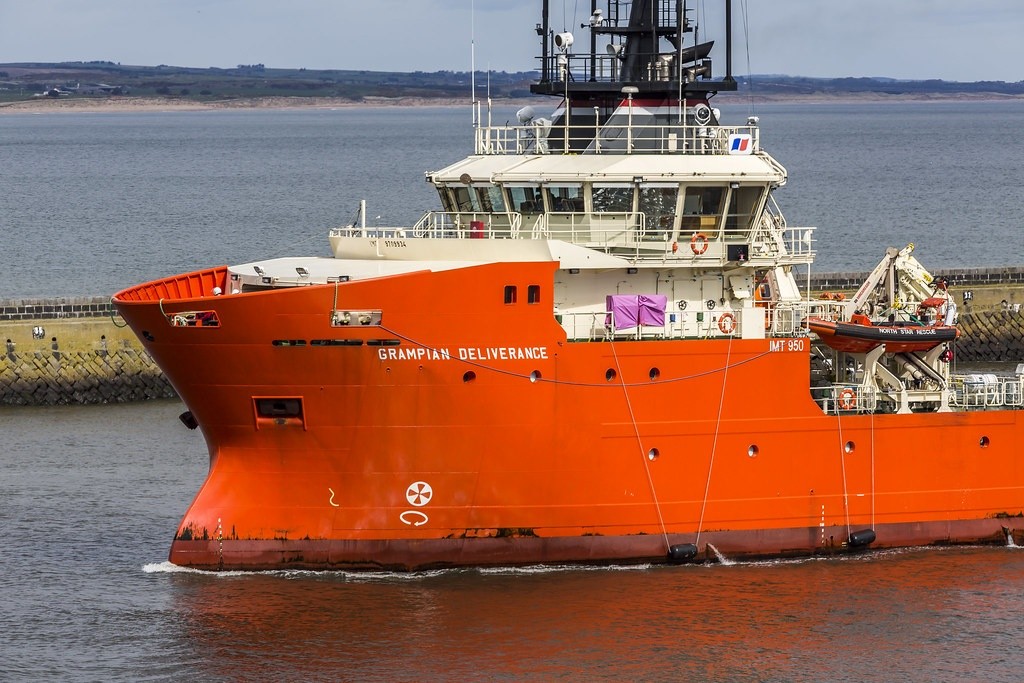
[839,389,857,411]
[691,234,709,254]
[719,313,737,333]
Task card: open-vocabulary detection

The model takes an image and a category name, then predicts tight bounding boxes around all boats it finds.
[802,314,961,353]
[110,1,1024,573]
